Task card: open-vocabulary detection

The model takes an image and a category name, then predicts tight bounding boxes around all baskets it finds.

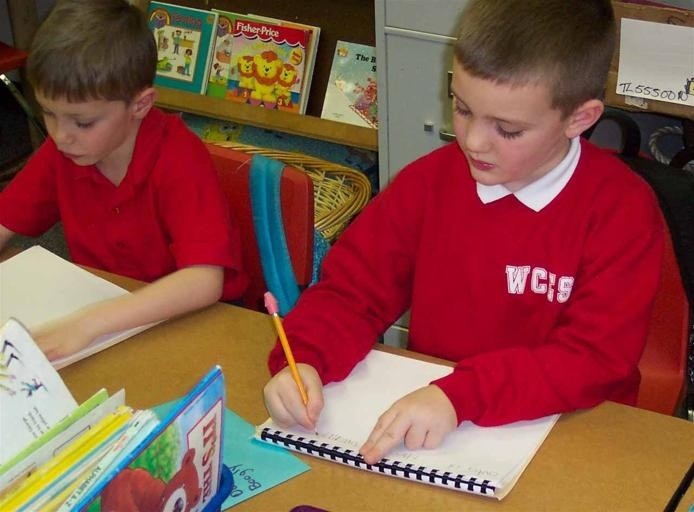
[201,140,372,243]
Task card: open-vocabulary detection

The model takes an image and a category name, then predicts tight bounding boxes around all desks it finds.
[0,246,694,512]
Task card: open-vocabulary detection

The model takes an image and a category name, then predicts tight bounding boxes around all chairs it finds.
[614,152,694,418]
[201,142,314,314]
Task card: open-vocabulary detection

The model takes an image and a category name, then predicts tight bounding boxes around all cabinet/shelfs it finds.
[374,0,470,349]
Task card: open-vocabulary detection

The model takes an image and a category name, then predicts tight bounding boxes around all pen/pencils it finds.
[264,291,319,436]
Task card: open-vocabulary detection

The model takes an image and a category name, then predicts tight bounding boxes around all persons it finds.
[263,0,691,466]
[0,0,251,363]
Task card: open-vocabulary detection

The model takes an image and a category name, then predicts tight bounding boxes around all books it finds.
[2,317,225,512]
[253,350,561,501]
